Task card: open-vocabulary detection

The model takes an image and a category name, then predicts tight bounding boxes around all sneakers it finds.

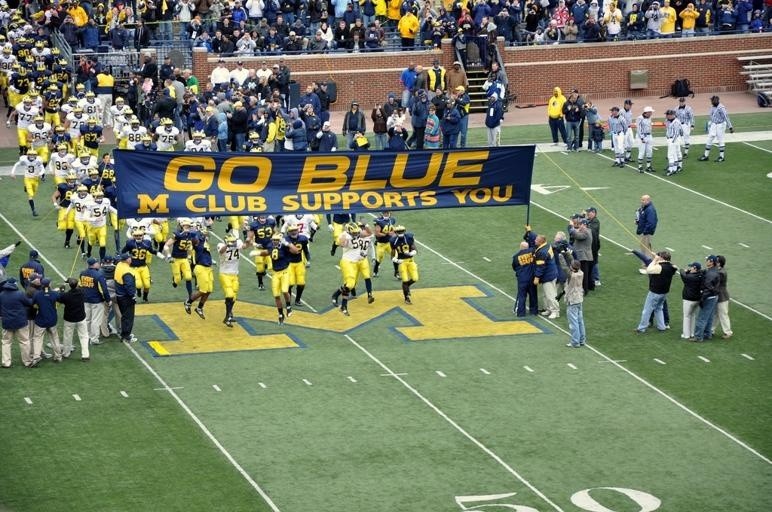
[394,274,402,281]
[258,284,265,291]
[351,296,358,299]
[714,156,724,162]
[128,333,135,339]
[404,297,411,304]
[278,315,284,326]
[679,333,691,339]
[541,310,550,316]
[665,170,676,177]
[612,161,619,168]
[673,167,684,175]
[548,311,560,319]
[64,243,70,248]
[632,328,648,335]
[619,161,625,168]
[638,166,644,173]
[223,318,233,327]
[682,154,688,160]
[330,241,337,257]
[229,317,236,322]
[688,336,704,343]
[698,155,709,161]
[372,272,380,278]
[565,342,580,347]
[330,294,339,309]
[29,357,43,368]
[294,300,304,306]
[339,304,351,317]
[63,351,71,356]
[287,310,293,317]
[195,307,206,320]
[43,354,53,359]
[721,331,735,340]
[122,337,138,345]
[646,167,657,172]
[368,297,374,304]
[183,299,192,315]
[627,156,636,163]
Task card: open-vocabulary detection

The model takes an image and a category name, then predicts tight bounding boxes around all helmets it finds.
[10,12,22,20]
[192,216,206,230]
[77,183,88,191]
[128,118,140,124]
[22,96,32,102]
[191,130,206,139]
[85,91,96,97]
[345,222,361,238]
[34,40,45,48]
[115,96,125,102]
[286,225,298,232]
[88,167,99,176]
[24,55,34,63]
[34,113,44,121]
[393,225,406,232]
[26,148,37,154]
[87,115,97,122]
[2,46,13,53]
[67,95,78,101]
[11,60,21,68]
[130,226,146,236]
[37,63,47,71]
[91,185,102,195]
[51,47,60,55]
[178,217,193,227]
[248,130,259,139]
[65,170,77,179]
[224,233,237,246]
[55,143,67,151]
[72,103,82,111]
[18,37,26,42]
[0,34,5,39]
[15,19,26,25]
[159,117,174,125]
[58,59,67,65]
[122,106,132,113]
[270,234,281,240]
[47,83,59,91]
[79,150,91,157]
[76,83,86,90]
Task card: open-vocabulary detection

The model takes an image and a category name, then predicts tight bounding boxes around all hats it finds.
[387,92,396,99]
[570,213,579,219]
[351,101,359,106]
[706,254,718,263]
[578,219,588,225]
[624,99,634,104]
[709,95,719,102]
[119,251,133,260]
[609,106,620,111]
[323,121,330,131]
[87,257,98,265]
[678,97,686,102]
[569,259,581,269]
[29,250,38,259]
[453,61,461,65]
[585,207,597,214]
[664,109,676,115]
[40,278,50,285]
[102,255,113,261]
[687,261,701,270]
[569,89,579,94]
[643,106,655,114]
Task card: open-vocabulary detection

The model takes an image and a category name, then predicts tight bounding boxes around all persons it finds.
[211,58,231,92]
[184,129,211,152]
[228,60,250,86]
[634,106,657,174]
[331,222,373,316]
[423,103,440,149]
[112,109,138,149]
[371,102,388,151]
[576,206,601,287]
[98,253,122,337]
[116,119,148,150]
[565,212,583,261]
[277,58,290,112]
[163,217,193,300]
[387,107,415,151]
[316,81,330,123]
[425,58,446,105]
[453,86,471,148]
[5,99,40,156]
[242,68,260,88]
[160,55,176,88]
[0,0,72,100]
[482,72,505,100]
[63,1,770,63]
[268,63,290,98]
[215,112,228,152]
[129,52,159,90]
[78,257,114,345]
[126,201,363,260]
[187,74,246,131]
[591,119,605,154]
[98,152,115,190]
[103,177,125,258]
[634,195,658,269]
[255,60,273,82]
[121,229,169,304]
[26,115,54,184]
[383,92,400,116]
[389,225,420,305]
[203,107,219,152]
[76,116,104,162]
[64,145,101,191]
[567,218,593,296]
[583,101,601,152]
[133,136,158,151]
[341,100,366,151]
[25,267,54,360]
[373,211,403,281]
[512,242,539,316]
[572,89,585,149]
[32,277,64,368]
[662,108,680,177]
[412,89,433,149]
[441,98,461,149]
[298,85,321,120]
[277,96,307,128]
[129,66,186,131]
[605,107,628,168]
[664,97,695,159]
[216,220,252,330]
[348,130,370,151]
[41,90,107,128]
[406,88,418,119]
[93,66,115,128]
[619,99,637,163]
[11,149,45,217]
[431,86,448,121]
[711,255,734,340]
[525,225,559,319]
[285,120,307,151]
[114,254,139,343]
[152,117,180,151]
[183,68,199,97]
[50,126,73,152]
[546,87,569,147]
[52,169,80,249]
[19,250,45,289]
[552,231,573,301]
[409,65,426,104]
[686,255,721,342]
[400,62,418,111]
[107,97,130,147]
[488,61,508,91]
[249,232,301,326]
[0,277,35,368]
[183,215,215,320]
[63,183,93,259]
[315,120,338,152]
[75,51,101,98]
[310,80,324,93]
[247,90,285,153]
[227,101,247,152]
[49,145,75,186]
[697,95,735,161]
[561,93,584,153]
[446,62,469,98]
[554,244,587,348]
[243,213,278,291]
[672,261,706,339]
[82,192,119,259]
[300,104,321,151]
[633,251,675,336]
[485,93,503,146]
[632,248,677,330]
[58,277,93,362]
